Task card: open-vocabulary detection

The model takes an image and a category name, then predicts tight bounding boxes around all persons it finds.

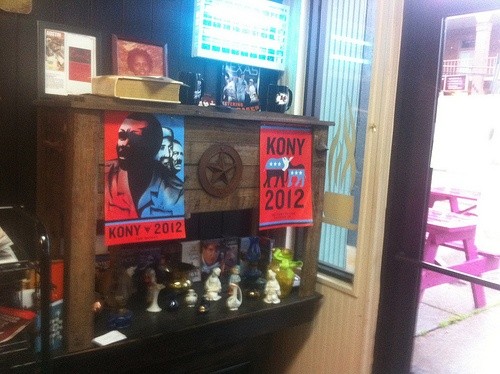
[118,254,179,311]
[204,267,223,301]
[201,240,221,277]
[127,48,154,77]
[224,71,258,103]
[263,269,282,303]
[228,264,241,295]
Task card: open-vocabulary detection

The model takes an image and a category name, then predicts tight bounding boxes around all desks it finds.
[0,276,323,374]
[32,97,335,354]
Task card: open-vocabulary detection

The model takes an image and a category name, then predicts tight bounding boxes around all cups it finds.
[272,248,294,263]
[266,85,294,113]
[178,72,206,106]
[271,261,297,297]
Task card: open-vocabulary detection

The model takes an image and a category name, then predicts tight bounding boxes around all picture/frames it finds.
[37,20,103,100]
[111,32,168,77]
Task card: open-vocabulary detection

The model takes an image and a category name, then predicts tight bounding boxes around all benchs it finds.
[429,186,477,216]
[421,208,487,309]
